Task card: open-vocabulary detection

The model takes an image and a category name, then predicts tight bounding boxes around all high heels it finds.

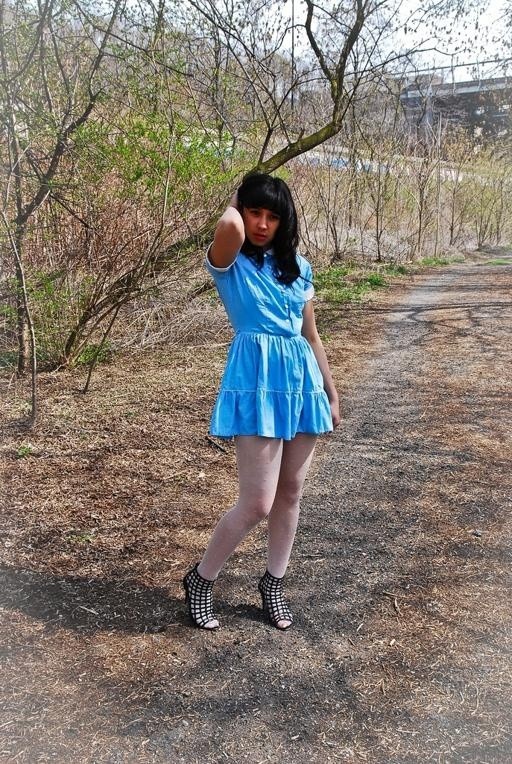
[183,562,220,630]
[258,567,293,630]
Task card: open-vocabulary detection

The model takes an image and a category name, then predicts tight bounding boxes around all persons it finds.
[176,169,342,632]
